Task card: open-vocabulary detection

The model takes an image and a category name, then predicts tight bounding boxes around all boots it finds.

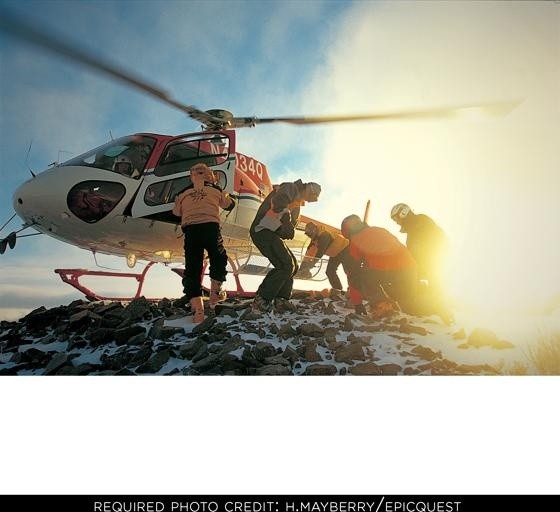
[208,278,228,310]
[189,296,205,323]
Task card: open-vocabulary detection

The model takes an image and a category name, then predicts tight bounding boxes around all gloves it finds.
[354,303,367,316]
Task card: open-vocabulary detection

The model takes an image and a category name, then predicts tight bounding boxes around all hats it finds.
[189,163,216,184]
[305,222,317,237]
[309,181,322,202]
[341,215,360,240]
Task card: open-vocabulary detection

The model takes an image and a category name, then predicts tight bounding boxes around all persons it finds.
[112,154,154,197]
[172,162,237,324]
[249,177,322,310]
[338,214,416,315]
[390,201,453,279]
[300,218,352,290]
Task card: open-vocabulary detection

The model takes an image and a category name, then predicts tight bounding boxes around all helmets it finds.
[390,203,412,221]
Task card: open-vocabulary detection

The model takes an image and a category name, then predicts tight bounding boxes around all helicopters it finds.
[0,6,523,303]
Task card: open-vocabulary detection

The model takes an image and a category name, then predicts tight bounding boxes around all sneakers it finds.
[253,294,277,315]
[274,296,296,313]
